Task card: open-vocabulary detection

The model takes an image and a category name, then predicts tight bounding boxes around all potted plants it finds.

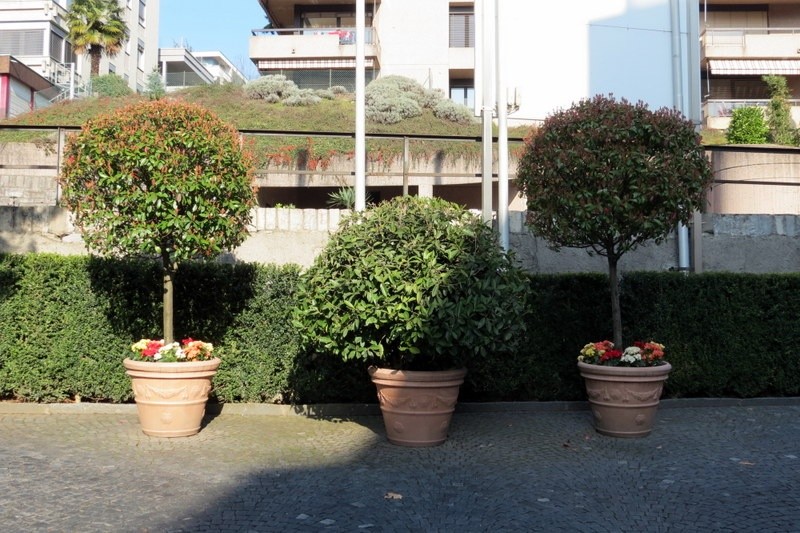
[294,202,533,446]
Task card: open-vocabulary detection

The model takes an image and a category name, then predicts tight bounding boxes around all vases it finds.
[576,357,676,436]
[123,355,225,438]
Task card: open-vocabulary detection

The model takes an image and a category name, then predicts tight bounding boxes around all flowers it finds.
[515,93,717,368]
[59,94,263,363]
